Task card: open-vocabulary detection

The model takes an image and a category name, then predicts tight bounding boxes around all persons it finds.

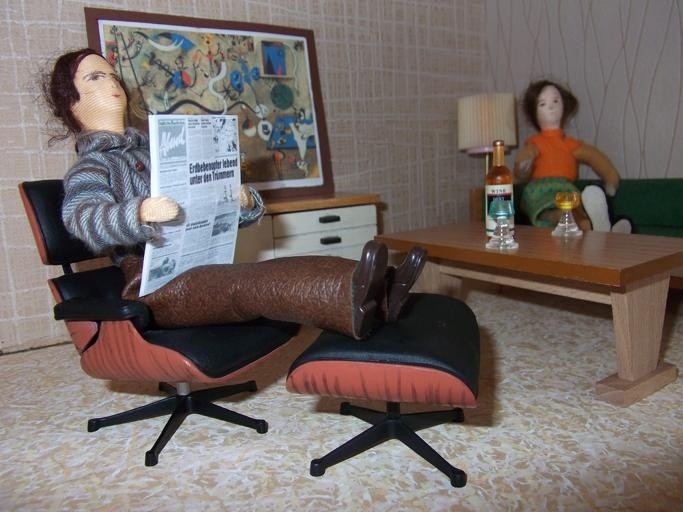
[30,49,429,344]
[518,78,631,235]
